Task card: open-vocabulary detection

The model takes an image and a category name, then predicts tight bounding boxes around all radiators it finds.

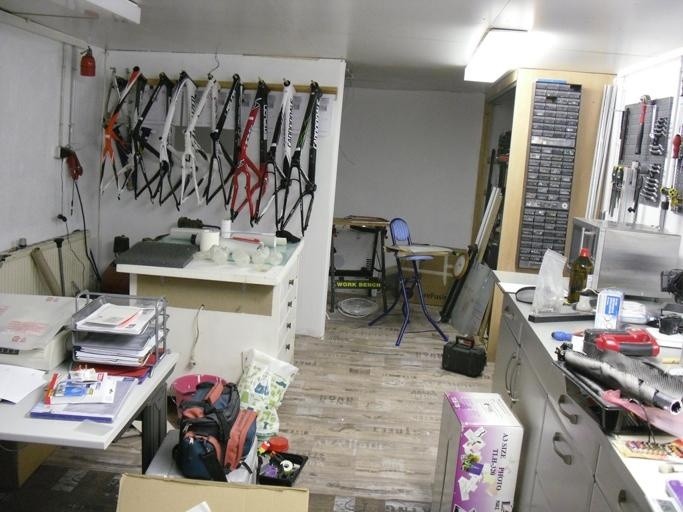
[0,230,89,298]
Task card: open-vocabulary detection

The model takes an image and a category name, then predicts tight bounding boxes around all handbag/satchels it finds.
[181,379,257,480]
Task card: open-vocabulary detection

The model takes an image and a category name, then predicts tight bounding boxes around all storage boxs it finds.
[428,389,525,511]
[398,245,467,307]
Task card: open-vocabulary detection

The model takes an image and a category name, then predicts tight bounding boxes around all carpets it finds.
[1,461,433,512]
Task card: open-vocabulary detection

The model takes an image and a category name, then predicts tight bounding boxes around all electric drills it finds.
[594,322,660,357]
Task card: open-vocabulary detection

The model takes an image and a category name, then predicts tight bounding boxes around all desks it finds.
[329,217,390,314]
[0,352,179,478]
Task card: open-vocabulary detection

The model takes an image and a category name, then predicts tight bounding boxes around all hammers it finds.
[634,94,651,155]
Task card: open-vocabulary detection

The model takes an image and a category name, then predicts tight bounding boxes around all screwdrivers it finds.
[609,165,622,217]
[670,134,681,188]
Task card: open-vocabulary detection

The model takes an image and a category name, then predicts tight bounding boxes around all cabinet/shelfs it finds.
[115,228,304,397]
[469,66,618,364]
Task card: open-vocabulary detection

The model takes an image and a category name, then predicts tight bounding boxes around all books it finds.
[67,304,165,383]
[26,376,139,423]
[44,370,108,404]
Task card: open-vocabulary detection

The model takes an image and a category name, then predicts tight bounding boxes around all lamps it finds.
[79,45,96,77]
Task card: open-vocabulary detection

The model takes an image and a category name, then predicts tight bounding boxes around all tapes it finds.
[279,459,293,472]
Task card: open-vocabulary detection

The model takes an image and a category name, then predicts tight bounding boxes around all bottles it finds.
[566,247,595,304]
